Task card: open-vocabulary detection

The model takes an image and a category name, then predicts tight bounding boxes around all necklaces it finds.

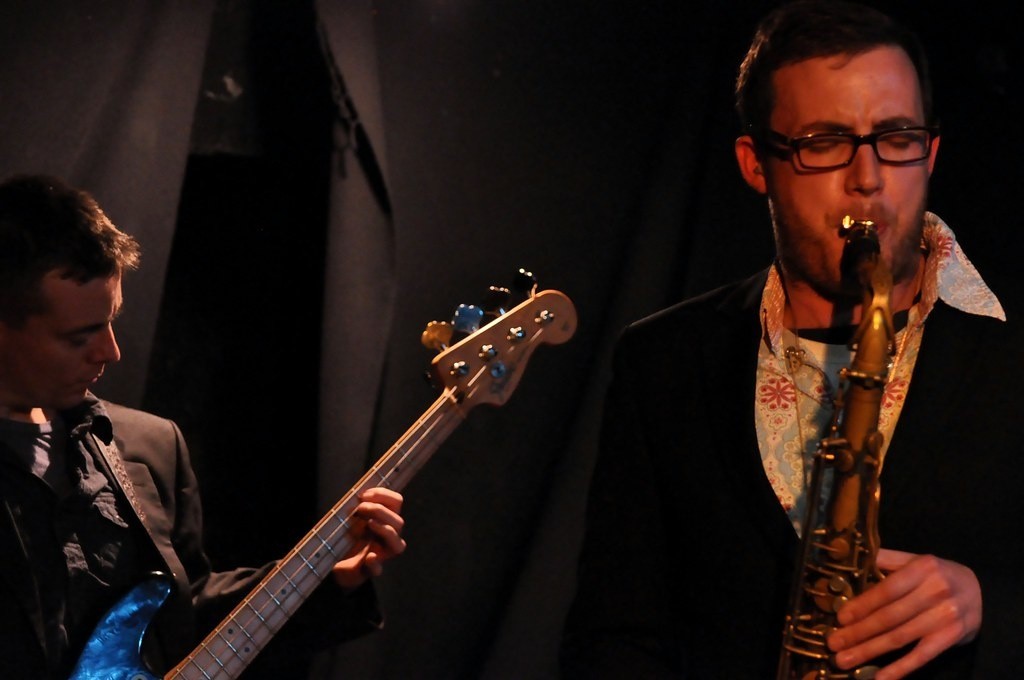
[786,256,924,496]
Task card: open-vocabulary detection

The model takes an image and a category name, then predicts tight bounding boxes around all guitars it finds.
[70,266,580,680]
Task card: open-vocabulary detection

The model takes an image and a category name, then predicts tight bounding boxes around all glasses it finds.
[748,124,942,170]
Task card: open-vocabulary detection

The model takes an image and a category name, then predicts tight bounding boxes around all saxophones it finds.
[775,214,898,680]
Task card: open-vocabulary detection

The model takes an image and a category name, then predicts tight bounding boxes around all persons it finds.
[0,175,406,680]
[557,0,1024,680]
